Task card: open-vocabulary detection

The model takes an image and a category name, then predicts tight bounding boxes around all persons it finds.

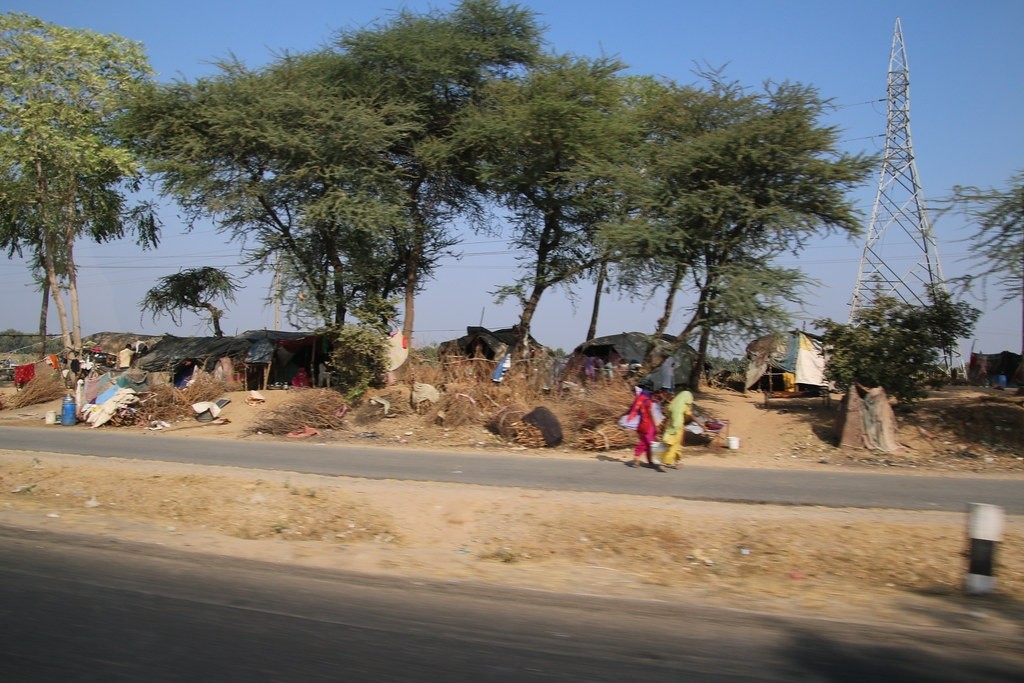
[626,387,666,468]
[659,391,707,472]
[583,356,641,386]
[117,343,142,370]
[174,357,195,387]
[291,367,310,387]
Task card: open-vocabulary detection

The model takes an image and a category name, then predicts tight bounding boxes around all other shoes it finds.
[675,463,685,471]
[648,462,659,468]
[657,463,667,472]
[631,460,640,467]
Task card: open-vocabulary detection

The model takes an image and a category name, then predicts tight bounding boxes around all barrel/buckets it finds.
[61,403,76,425]
[727,437,740,450]
[46,411,56,424]
[649,441,668,464]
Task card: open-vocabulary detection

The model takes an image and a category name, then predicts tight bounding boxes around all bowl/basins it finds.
[267,384,282,389]
